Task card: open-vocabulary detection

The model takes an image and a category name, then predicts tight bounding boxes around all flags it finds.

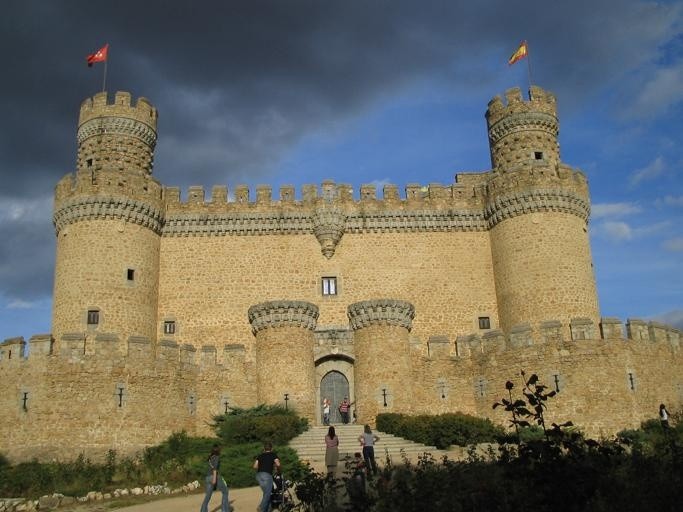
[507,40,528,66]
[85,43,107,67]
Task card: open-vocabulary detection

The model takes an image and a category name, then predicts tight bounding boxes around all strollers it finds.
[271,473,295,510]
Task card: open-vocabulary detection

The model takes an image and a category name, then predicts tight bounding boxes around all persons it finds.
[252,442,281,512]
[324,426,340,481]
[339,399,351,424]
[659,403,670,433]
[199,444,231,512]
[322,399,331,426]
[357,424,380,481]
